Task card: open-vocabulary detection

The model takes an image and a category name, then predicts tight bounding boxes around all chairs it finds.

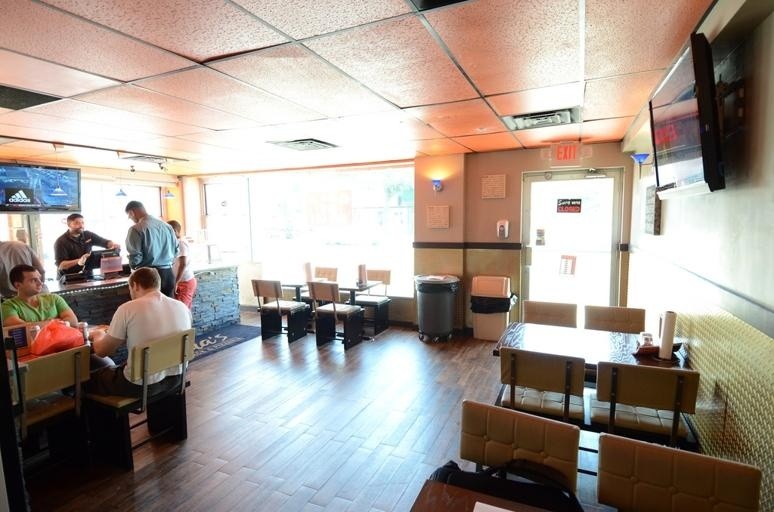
[499,345,584,423]
[597,430,762,512]
[589,362,701,447]
[86,331,201,471]
[522,299,577,328]
[17,345,92,478]
[584,305,646,334]
[250,262,391,351]
[461,396,580,498]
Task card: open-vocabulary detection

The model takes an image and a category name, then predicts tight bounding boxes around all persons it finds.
[0,241,47,297]
[124,200,178,298]
[15,229,28,245]
[0,265,80,331]
[53,214,122,281]
[80,266,194,398]
[166,220,196,310]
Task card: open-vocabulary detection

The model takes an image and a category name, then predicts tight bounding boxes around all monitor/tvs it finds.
[0,162,81,212]
[646,33,725,201]
[82,249,120,279]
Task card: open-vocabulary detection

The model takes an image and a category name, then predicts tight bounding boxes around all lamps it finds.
[116,187,125,196]
[49,143,68,196]
[130,164,137,178]
[630,151,655,181]
[432,180,443,192]
[164,187,175,198]
[159,162,168,174]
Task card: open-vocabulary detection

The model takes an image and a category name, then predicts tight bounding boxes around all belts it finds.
[152,265,171,269]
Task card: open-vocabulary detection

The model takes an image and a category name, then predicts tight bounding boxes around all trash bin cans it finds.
[415,274,459,344]
[471,275,512,342]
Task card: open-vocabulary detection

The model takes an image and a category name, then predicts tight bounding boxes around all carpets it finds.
[186,324,262,363]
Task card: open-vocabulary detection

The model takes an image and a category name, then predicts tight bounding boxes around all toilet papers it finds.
[658,311,677,361]
[357,265,367,288]
[304,262,314,284]
